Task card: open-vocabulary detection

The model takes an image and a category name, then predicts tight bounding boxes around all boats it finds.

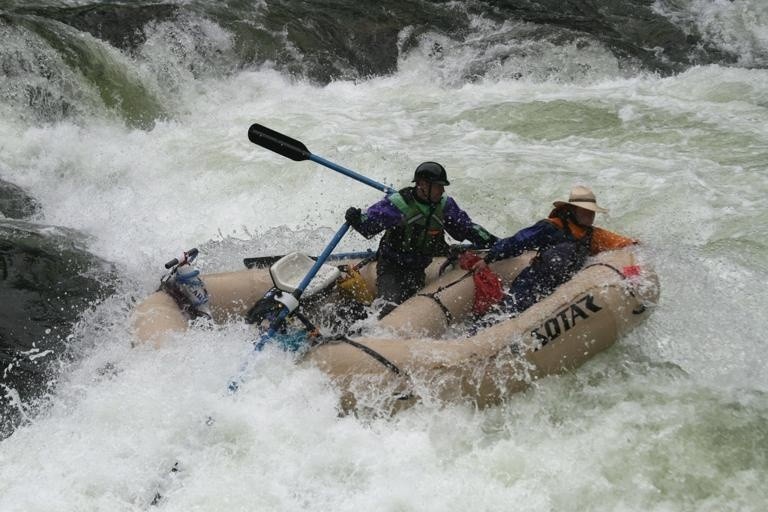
[131,227,664,423]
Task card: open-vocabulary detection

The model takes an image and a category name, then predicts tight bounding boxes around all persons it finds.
[344,161,499,320]
[470,184,641,335]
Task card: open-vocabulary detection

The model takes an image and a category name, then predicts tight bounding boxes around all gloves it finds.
[343,206,363,229]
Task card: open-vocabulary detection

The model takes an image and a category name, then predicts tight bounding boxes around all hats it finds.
[551,184,611,214]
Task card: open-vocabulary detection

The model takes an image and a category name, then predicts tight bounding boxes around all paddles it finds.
[243,252,375,269]
[248,124,396,194]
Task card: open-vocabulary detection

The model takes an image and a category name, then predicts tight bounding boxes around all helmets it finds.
[411,160,451,187]
